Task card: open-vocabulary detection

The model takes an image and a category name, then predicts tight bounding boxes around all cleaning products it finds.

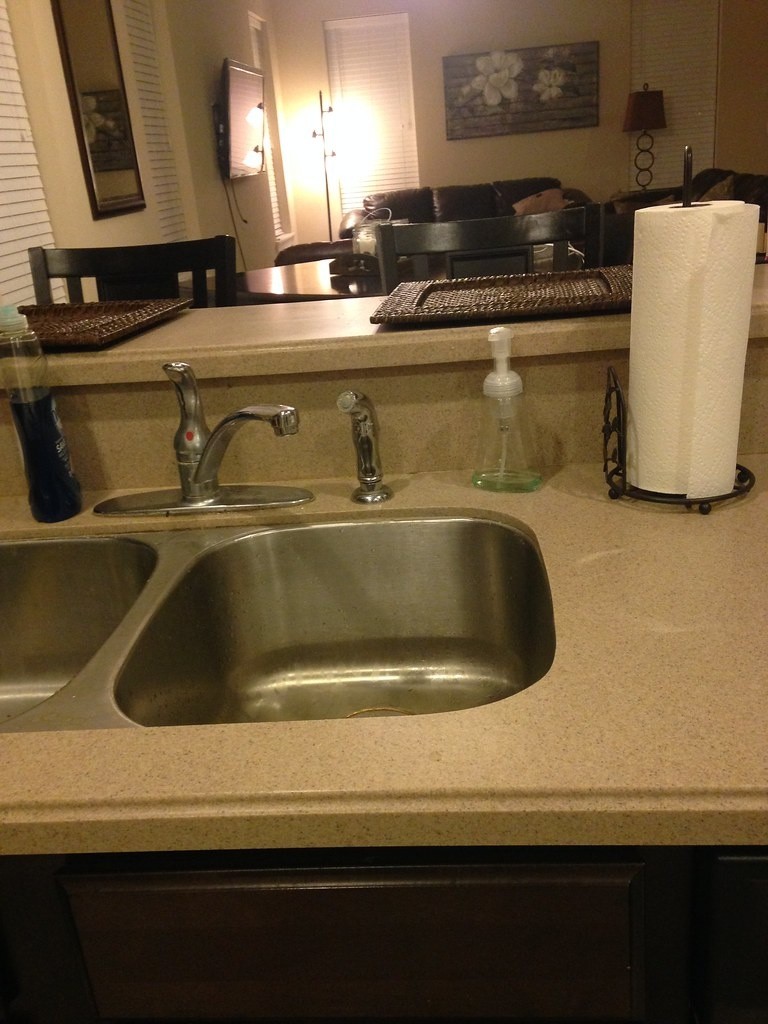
[0,301,85,524]
[472,326,545,495]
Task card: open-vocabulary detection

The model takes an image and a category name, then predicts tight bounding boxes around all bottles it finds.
[0,313,84,523]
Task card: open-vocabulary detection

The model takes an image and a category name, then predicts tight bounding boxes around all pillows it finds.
[697,174,735,202]
[613,194,675,216]
[513,189,575,217]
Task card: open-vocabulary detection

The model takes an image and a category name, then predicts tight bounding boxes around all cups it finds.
[340,259,370,295]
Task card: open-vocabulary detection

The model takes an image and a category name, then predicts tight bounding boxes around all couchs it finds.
[601,168,768,267]
[339,176,593,240]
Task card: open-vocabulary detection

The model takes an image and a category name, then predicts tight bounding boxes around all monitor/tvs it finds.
[219,56,267,179]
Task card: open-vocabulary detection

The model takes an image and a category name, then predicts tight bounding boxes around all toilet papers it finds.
[623,199,762,500]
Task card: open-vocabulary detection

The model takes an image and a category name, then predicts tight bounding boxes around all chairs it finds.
[28,233,239,311]
[374,207,587,295]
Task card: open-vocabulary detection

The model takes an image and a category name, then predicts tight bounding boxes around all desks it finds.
[180,257,387,308]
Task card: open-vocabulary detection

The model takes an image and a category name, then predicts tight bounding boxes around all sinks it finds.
[0,536,161,727]
[110,514,559,728]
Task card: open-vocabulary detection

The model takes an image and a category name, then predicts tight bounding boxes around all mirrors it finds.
[50,0,147,222]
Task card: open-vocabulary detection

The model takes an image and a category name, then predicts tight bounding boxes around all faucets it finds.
[161,360,303,500]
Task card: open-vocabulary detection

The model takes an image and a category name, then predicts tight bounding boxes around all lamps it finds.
[622,82,668,194]
